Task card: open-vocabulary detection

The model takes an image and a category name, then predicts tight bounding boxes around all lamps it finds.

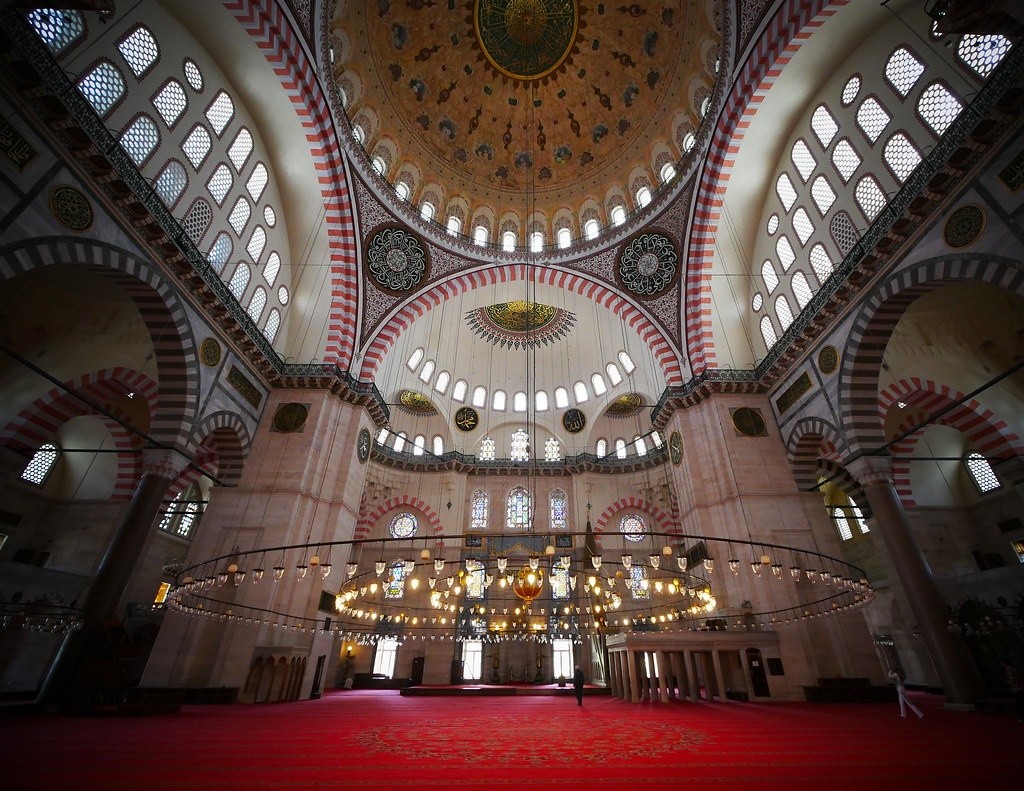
[0,601,88,636]
[946,605,1024,638]
[166,531,876,645]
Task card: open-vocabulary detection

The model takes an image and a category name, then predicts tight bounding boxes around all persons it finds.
[888,663,928,720]
[573,663,585,707]
